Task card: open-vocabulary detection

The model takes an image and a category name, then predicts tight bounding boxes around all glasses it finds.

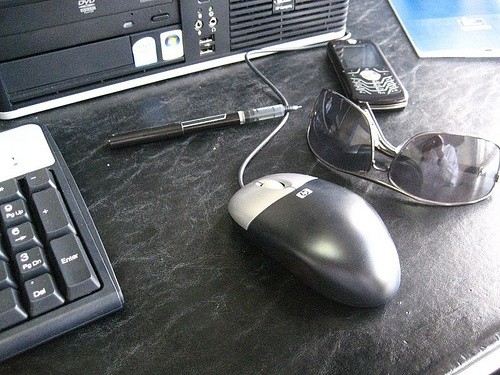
[306,87,500,207]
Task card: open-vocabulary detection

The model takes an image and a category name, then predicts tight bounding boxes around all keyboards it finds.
[0,122,125,365]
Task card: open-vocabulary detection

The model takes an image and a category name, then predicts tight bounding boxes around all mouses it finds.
[228,172,399,310]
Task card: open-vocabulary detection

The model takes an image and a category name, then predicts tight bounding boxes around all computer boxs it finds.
[0,0,347,122]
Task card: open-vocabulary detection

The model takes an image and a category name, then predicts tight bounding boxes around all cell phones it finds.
[327,37,408,110]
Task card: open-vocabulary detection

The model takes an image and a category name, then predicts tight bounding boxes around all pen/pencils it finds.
[106,103,303,148]
